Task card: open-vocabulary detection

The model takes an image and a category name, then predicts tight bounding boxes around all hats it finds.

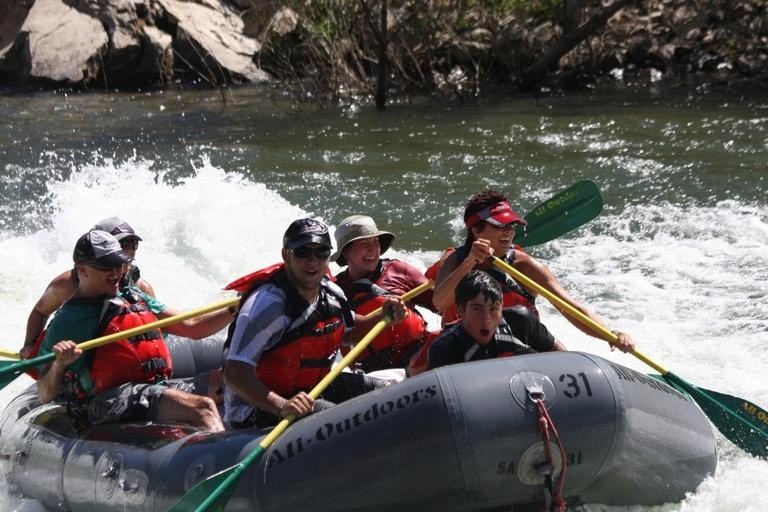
[328,215,397,267]
[73,228,136,266]
[283,218,333,249]
[466,201,527,228]
[93,217,142,242]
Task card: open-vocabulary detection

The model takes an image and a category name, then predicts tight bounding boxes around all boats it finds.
[0,332,718,511]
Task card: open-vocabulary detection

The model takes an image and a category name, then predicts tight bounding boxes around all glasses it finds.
[290,246,331,260]
[120,239,139,251]
[88,262,122,271]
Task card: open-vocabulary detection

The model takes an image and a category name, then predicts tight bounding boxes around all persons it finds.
[37,229,242,432]
[405,269,568,385]
[19,215,157,381]
[431,188,636,354]
[221,218,409,434]
[328,215,442,375]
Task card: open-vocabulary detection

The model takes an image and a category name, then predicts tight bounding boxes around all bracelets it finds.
[279,398,284,420]
[228,306,238,318]
[23,341,35,346]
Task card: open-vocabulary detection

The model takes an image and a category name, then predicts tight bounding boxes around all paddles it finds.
[365,181,603,319]
[169,305,396,512]
[487,254,768,460]
